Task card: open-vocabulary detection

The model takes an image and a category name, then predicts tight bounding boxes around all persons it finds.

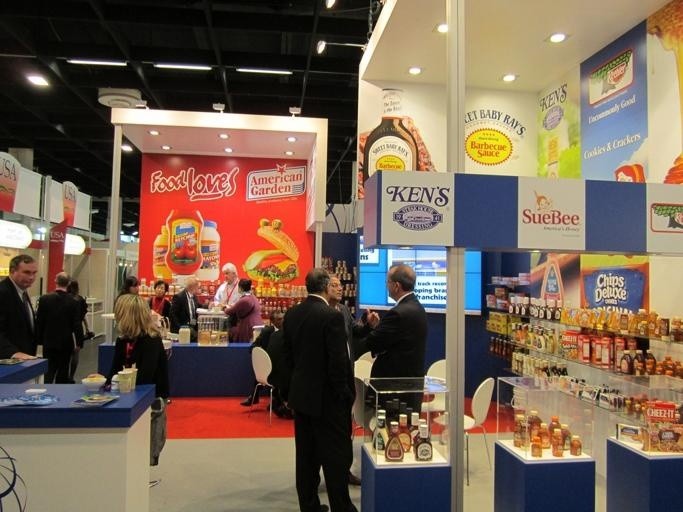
[277,269,382,512]
[0,254,39,361]
[360,263,427,429]
[66,279,88,385]
[240,310,293,419]
[35,271,82,384]
[112,276,171,465]
[167,262,264,344]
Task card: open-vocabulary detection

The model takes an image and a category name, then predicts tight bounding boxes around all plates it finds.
[2,393,60,407]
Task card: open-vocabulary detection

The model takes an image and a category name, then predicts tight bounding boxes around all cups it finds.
[119,367,139,393]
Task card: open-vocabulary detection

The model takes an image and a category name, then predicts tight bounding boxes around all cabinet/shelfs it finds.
[483,294,683,494]
[82,298,106,340]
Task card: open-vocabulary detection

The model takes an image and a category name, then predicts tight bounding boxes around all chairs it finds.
[351,351,377,444]
[433,378,495,487]
[420,360,447,445]
[247,347,286,426]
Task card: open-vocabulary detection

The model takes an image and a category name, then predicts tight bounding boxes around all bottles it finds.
[364,394,381,434]
[362,89,420,186]
[178,325,191,345]
[622,391,682,425]
[616,349,683,384]
[638,309,683,343]
[512,411,584,457]
[510,296,567,392]
[375,400,433,462]
[251,277,307,316]
[111,375,119,393]
[541,252,566,310]
[152,207,221,287]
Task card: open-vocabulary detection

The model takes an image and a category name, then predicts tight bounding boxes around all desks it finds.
[0,357,157,512]
[97,342,272,397]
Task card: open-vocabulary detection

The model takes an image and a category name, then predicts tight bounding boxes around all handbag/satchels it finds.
[227,314,236,328]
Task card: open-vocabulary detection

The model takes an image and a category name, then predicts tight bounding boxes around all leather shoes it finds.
[266,399,293,418]
[239,394,259,406]
[348,469,362,485]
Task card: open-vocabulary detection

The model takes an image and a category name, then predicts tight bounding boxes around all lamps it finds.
[325,0,388,10]
[66,59,128,67]
[289,107,301,116]
[152,62,213,72]
[134,100,149,109]
[235,67,293,75]
[212,102,226,112]
[317,40,368,55]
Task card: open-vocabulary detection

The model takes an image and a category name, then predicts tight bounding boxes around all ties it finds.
[23,292,30,318]
[191,298,195,320]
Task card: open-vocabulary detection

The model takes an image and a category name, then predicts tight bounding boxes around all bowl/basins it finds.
[82,378,108,391]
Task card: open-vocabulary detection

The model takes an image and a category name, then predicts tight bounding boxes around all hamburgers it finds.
[243,218,298,284]
